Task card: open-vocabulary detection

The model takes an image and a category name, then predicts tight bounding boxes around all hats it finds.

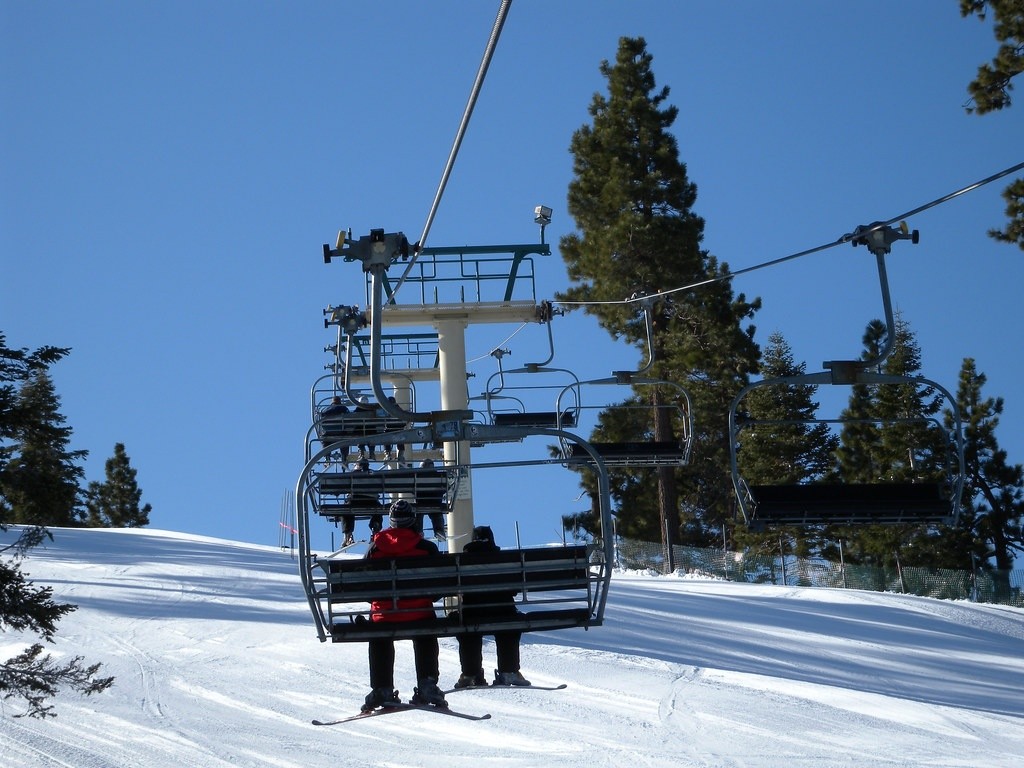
[389,499,415,528]
[471,526,495,542]
[359,396,370,403]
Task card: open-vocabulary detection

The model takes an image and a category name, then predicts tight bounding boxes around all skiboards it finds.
[357,456,382,466]
[308,464,355,480]
[320,537,373,558]
[417,531,476,543]
[375,459,412,470]
[442,684,567,695]
[311,703,492,727]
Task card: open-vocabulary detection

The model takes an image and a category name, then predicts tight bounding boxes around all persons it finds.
[361,499,447,711]
[455,526,532,688]
[354,396,377,461]
[340,457,383,548]
[409,459,446,542]
[321,396,352,468]
[383,396,406,464]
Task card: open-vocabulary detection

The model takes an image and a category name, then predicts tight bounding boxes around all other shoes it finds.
[454,670,486,688]
[323,460,330,467]
[418,684,445,704]
[498,671,532,686]
[365,688,395,707]
[434,532,446,542]
[342,461,348,468]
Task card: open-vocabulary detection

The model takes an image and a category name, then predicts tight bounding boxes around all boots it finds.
[398,450,406,464]
[370,527,379,543]
[341,532,354,547]
[368,450,375,460]
[356,450,365,461]
[383,450,393,462]
